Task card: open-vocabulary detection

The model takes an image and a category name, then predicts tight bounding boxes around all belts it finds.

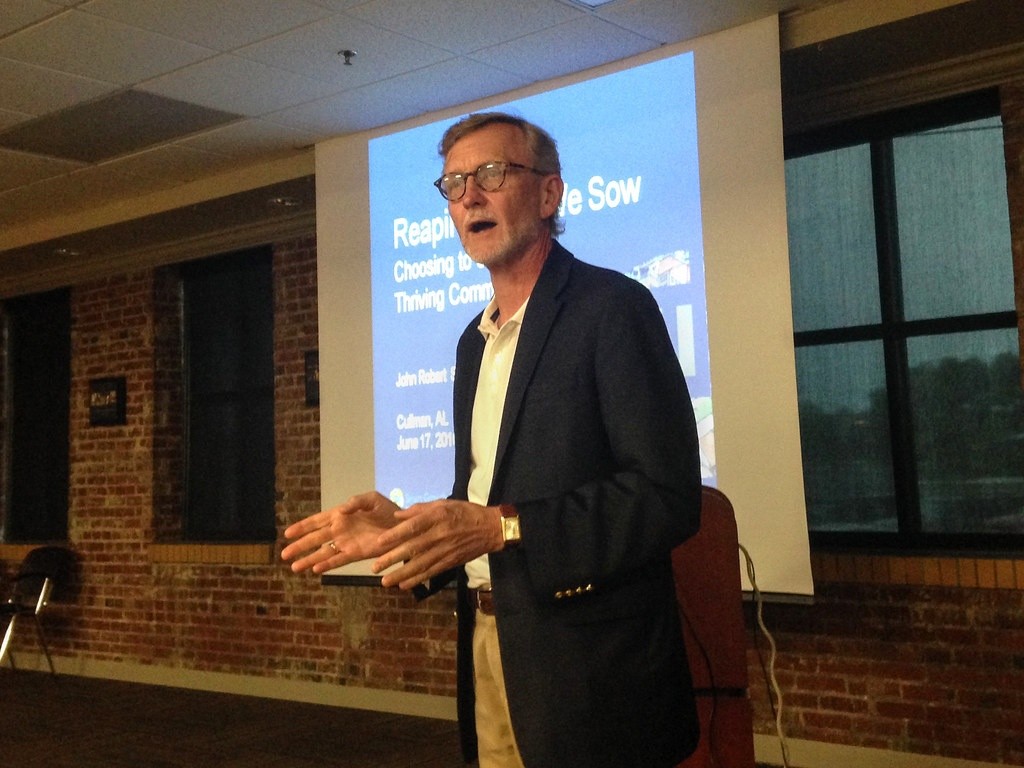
[469,589,496,615]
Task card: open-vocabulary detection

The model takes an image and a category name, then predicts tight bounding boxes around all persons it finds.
[280,111,702,768]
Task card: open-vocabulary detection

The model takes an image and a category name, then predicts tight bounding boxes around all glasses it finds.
[434,161,548,201]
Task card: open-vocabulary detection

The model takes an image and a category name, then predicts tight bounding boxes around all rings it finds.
[330,543,339,554]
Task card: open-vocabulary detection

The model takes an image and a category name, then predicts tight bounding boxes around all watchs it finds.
[500,504,521,545]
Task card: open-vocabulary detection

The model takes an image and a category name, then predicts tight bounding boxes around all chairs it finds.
[0,545,86,682]
